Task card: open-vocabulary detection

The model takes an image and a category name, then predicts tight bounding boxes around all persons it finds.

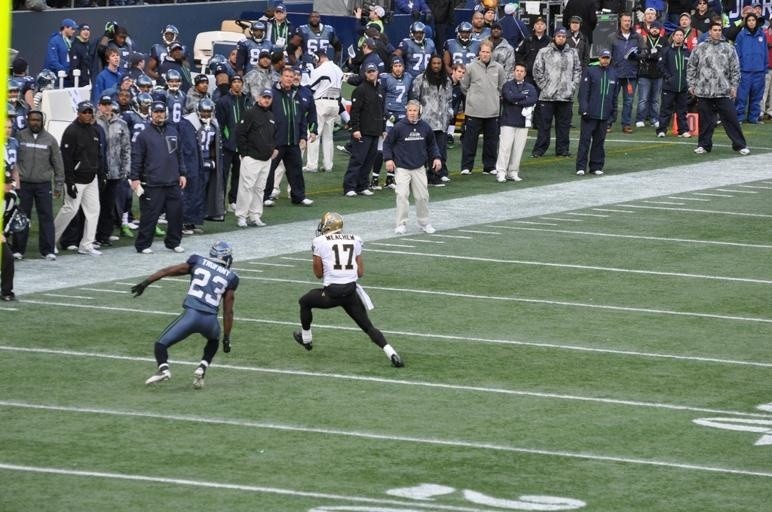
[0,160,19,302]
[130,242,241,389]
[1,2,346,261]
[561,1,772,176]
[343,1,586,196]
[293,211,406,368]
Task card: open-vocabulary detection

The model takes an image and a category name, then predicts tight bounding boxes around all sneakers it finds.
[144,369,172,388]
[390,355,405,368]
[302,166,318,174]
[318,166,332,172]
[15,183,313,259]
[336,146,351,155]
[592,171,603,176]
[396,218,411,236]
[737,148,753,159]
[344,149,571,198]
[192,368,207,389]
[0,290,19,304]
[575,169,586,176]
[417,221,437,235]
[603,115,770,139]
[693,144,712,157]
[291,331,315,352]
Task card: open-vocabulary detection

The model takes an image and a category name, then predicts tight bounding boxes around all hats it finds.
[364,39,403,72]
[76,69,243,114]
[62,18,80,29]
[130,53,144,65]
[78,24,91,31]
[260,48,327,75]
[276,6,286,13]
[5,69,57,124]
[261,89,272,97]
[368,5,385,18]
[489,1,763,58]
[166,41,183,55]
[365,28,380,40]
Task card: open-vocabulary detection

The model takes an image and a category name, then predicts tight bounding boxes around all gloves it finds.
[222,334,232,354]
[130,278,150,297]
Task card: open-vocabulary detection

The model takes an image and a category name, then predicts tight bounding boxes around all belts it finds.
[318,97,338,100]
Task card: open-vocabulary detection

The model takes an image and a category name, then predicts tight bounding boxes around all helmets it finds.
[251,22,265,42]
[456,21,474,47]
[315,211,344,235]
[208,54,228,72]
[209,240,234,262]
[410,20,428,43]
[105,21,120,34]
[160,24,179,44]
